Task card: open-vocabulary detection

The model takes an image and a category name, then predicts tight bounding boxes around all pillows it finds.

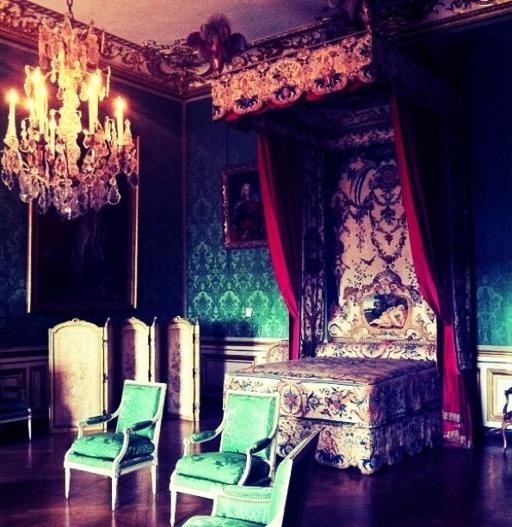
[314,343,436,362]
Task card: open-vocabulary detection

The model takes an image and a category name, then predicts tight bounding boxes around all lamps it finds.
[0,0,138,220]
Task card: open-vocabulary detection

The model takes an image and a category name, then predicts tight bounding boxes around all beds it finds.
[222,279,442,475]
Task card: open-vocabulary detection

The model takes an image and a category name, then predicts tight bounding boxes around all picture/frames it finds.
[221,163,270,249]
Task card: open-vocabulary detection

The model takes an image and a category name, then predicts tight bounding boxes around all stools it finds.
[0,384,32,441]
[502,388,512,455]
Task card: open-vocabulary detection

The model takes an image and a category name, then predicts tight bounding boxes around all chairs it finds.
[63,380,167,511]
[182,429,320,527]
[169,389,280,527]
